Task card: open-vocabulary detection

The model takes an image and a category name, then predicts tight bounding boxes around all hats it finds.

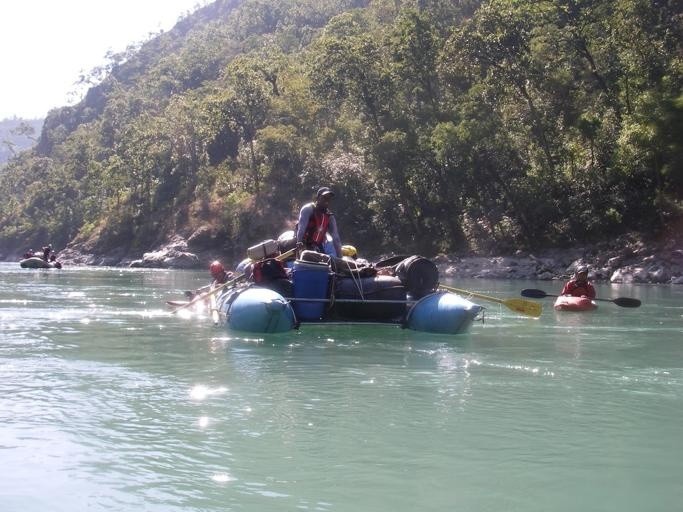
[577,266,588,274]
[317,187,334,197]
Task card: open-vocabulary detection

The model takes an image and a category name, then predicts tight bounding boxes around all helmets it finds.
[341,245,357,257]
[210,261,224,275]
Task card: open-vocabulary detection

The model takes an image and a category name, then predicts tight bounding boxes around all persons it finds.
[209,261,236,286]
[561,266,596,301]
[24,244,56,263]
[296,186,343,260]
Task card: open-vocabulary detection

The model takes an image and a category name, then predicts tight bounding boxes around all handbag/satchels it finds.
[254,259,287,283]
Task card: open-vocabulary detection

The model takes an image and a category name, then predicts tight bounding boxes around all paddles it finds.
[438,285,542,318]
[521,288,641,307]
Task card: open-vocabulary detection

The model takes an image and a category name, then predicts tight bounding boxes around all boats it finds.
[19,251,63,270]
[552,283,598,315]
[169,219,487,339]
